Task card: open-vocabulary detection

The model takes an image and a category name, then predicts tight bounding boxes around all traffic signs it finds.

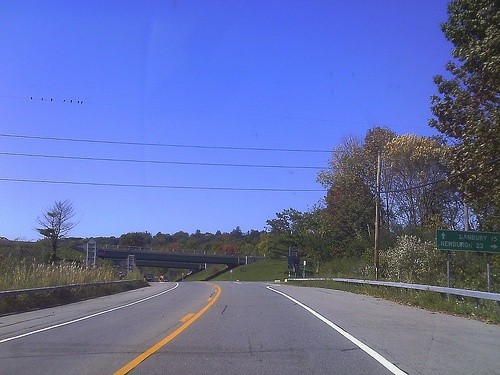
[435,229,500,255]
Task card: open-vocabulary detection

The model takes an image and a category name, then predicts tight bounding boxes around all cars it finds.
[145,273,154,281]
[158,276,168,281]
